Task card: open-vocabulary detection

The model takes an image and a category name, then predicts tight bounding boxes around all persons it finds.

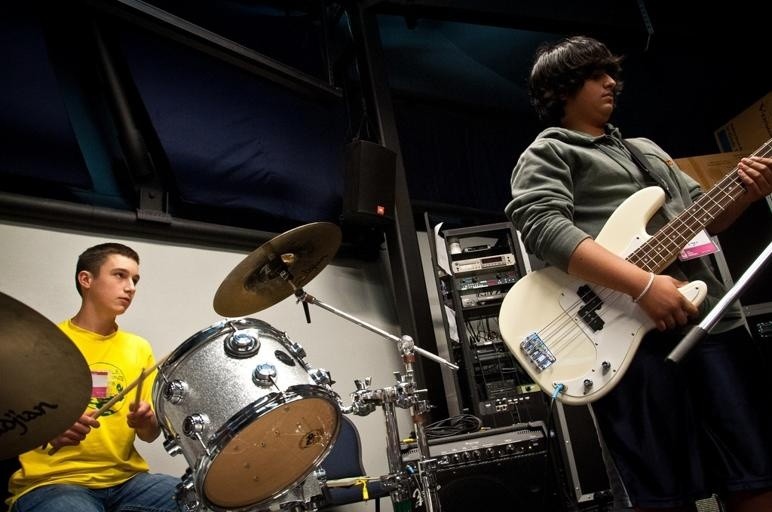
[504,31,771,512]
[3,243,209,512]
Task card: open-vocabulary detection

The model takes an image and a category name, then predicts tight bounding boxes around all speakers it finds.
[342,139,399,222]
[386,421,569,512]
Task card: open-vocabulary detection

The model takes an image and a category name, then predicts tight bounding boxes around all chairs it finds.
[314,414,395,512]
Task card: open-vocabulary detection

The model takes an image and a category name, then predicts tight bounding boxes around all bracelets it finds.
[632,269,655,303]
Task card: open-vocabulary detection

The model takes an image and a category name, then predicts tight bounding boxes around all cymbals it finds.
[214,221,343,318]
[0,292,93,459]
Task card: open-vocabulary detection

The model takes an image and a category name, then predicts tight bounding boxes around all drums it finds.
[152,317,343,504]
[172,468,329,511]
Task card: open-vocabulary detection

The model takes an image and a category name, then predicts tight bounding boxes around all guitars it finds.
[499,142,768,405]
